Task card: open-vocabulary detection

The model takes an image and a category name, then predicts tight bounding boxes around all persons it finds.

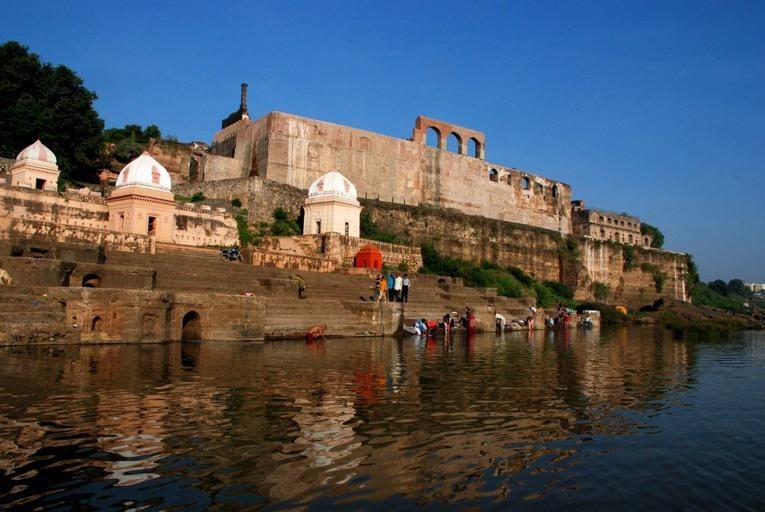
[221,248,240,262]
[547,304,573,329]
[373,271,410,303]
[584,315,591,326]
[289,273,306,298]
[496,304,536,332]
[305,324,328,341]
[414,304,475,336]
